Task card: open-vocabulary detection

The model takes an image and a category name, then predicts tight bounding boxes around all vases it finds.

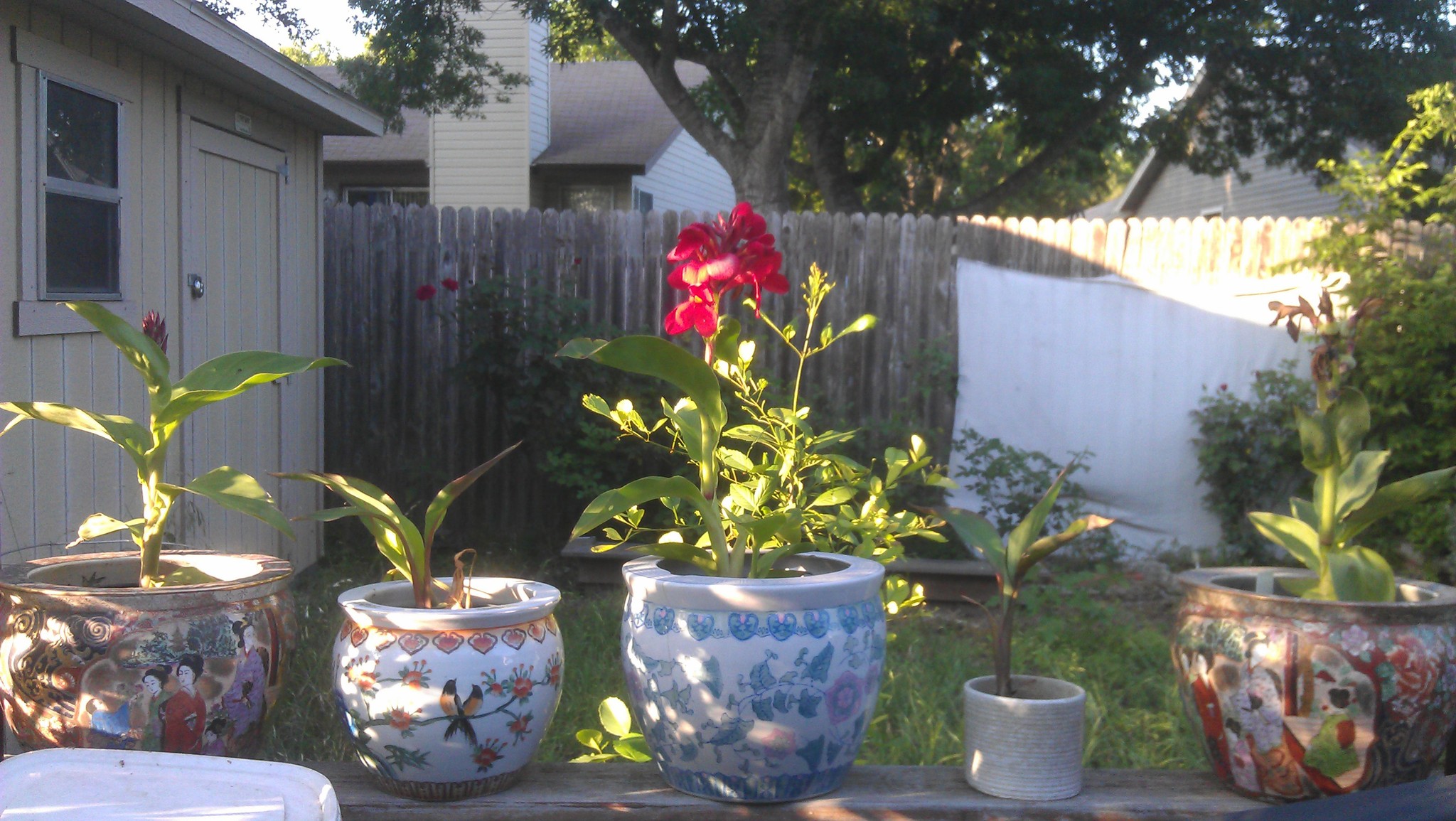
[620,547,890,803]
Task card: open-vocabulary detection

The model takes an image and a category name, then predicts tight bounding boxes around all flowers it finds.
[565,197,847,570]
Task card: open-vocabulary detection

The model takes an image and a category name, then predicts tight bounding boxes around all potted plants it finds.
[1169,288,1456,803]
[269,436,570,799]
[907,457,1114,801]
[1,272,358,754]
